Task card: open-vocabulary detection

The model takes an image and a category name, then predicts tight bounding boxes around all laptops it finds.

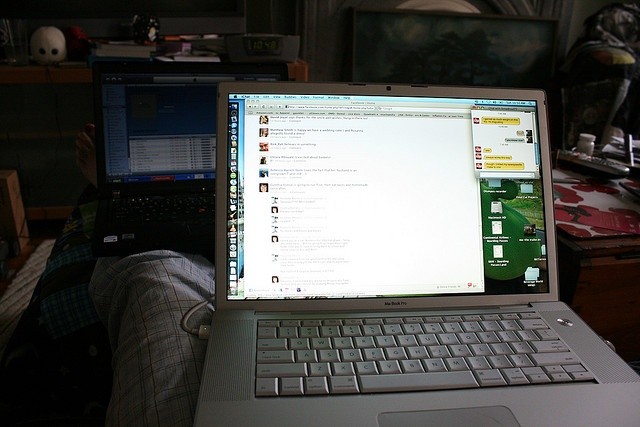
[87,57,288,258]
[192,82,640,427]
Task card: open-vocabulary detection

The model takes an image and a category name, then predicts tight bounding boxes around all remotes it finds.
[558,149,630,181]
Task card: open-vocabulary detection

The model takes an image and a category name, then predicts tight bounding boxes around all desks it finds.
[551,167,640,365]
